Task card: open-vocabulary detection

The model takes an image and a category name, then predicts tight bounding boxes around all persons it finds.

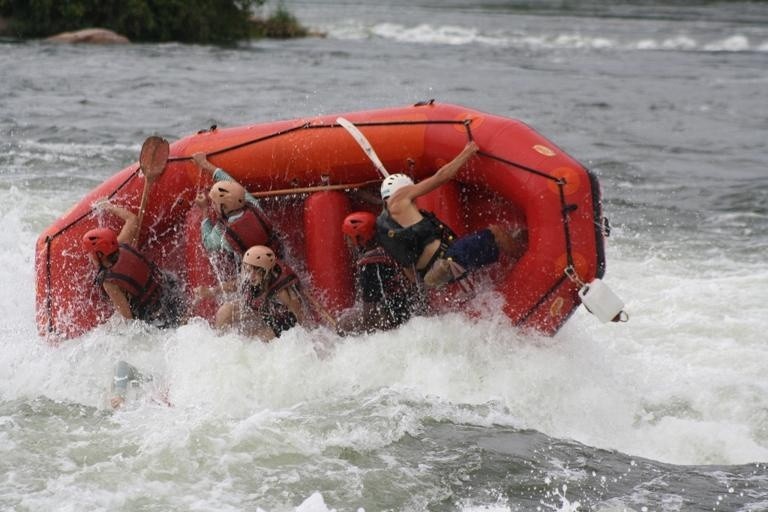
[81,199,163,319]
[375,142,524,283]
[193,246,306,340]
[192,150,283,261]
[334,211,408,335]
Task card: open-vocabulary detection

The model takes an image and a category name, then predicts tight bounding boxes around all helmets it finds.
[341,211,377,247]
[380,172,414,201]
[81,228,120,261]
[208,179,246,214]
[242,244,276,280]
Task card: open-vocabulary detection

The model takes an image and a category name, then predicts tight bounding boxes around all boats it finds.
[35,99,606,341]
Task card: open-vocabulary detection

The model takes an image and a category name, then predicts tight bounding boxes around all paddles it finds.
[130,135,170,255]
